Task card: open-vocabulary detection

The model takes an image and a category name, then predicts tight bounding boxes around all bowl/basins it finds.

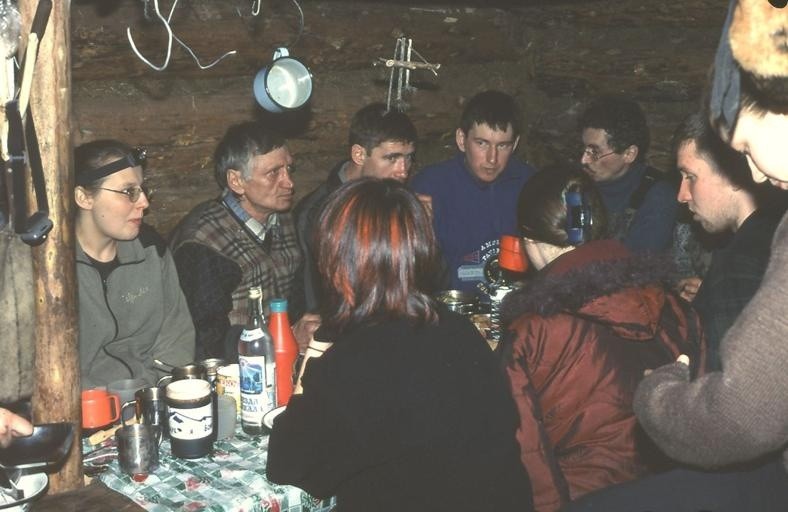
[1,469,49,511]
[1,421,77,473]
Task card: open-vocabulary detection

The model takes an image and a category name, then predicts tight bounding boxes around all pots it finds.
[434,289,481,316]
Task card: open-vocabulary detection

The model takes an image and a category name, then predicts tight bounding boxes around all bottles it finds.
[236,287,279,436]
[267,299,300,407]
[488,273,515,343]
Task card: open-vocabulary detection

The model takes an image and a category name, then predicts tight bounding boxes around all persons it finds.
[580,99,680,264]
[166,121,319,371]
[634,0,788,476]
[75,138,196,412]
[674,109,778,361]
[292,103,433,223]
[493,165,708,512]
[411,90,538,290]
[0,407,33,452]
[270,177,536,512]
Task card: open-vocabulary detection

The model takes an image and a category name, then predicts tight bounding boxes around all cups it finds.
[498,232,530,274]
[80,389,120,436]
[121,387,167,439]
[121,388,166,437]
[216,394,235,439]
[155,365,207,389]
[107,378,148,422]
[166,379,218,459]
[252,47,314,116]
[114,424,163,474]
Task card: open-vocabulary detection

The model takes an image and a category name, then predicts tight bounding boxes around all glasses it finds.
[93,181,153,201]
[586,150,617,162]
